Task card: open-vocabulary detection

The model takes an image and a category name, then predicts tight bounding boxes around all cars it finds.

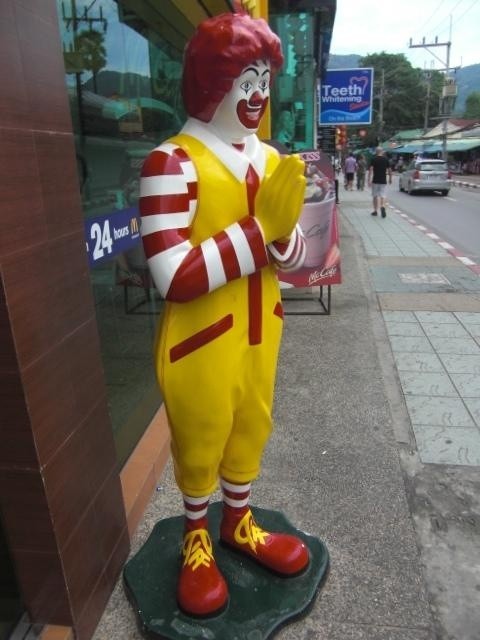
[73,96,184,192]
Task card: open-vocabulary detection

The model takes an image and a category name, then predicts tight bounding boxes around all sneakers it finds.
[371,212,378,216]
[380,206,386,218]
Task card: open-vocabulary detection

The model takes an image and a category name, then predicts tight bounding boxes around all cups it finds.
[298,197,336,268]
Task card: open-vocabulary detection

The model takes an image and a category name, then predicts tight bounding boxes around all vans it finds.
[399,156,452,196]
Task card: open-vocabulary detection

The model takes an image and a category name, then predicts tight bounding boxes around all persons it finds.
[139,13,308,621]
[340,146,421,220]
